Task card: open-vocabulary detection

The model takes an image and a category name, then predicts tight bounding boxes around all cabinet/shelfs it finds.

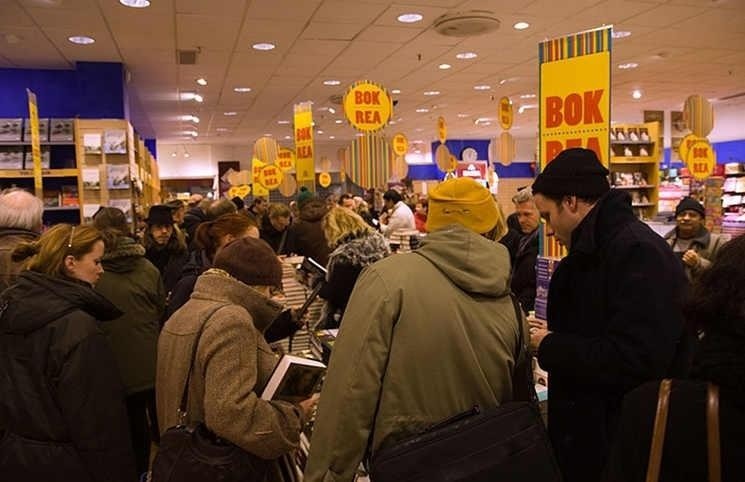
[0,117,133,237]
[132,130,161,223]
[611,121,662,221]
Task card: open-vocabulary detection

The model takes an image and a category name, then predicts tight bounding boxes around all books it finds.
[49,118,73,141]
[24,118,49,142]
[83,132,102,155]
[0,116,23,142]
[104,127,127,154]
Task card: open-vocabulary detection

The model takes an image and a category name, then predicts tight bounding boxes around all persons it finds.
[498,146,744,482]
[0,147,530,482]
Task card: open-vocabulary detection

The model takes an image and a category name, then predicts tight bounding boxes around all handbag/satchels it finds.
[370,401,563,482]
[151,421,270,482]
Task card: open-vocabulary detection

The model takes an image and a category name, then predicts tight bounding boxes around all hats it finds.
[298,185,314,210]
[675,197,704,218]
[532,148,610,193]
[214,237,282,286]
[425,178,498,234]
[144,205,176,224]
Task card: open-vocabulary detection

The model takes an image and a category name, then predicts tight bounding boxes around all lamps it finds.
[433,11,502,37]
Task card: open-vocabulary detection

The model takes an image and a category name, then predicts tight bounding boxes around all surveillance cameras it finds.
[392,98,399,107]
